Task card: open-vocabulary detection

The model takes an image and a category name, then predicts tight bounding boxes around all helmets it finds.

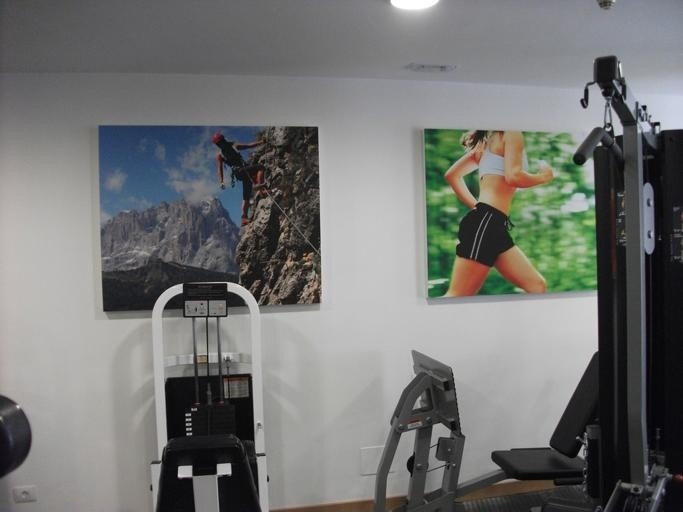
[212,132,224,146]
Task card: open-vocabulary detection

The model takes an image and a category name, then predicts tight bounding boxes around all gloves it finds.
[219,182,227,190]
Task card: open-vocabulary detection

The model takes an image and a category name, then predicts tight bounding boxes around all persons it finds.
[212,134,267,225]
[439,130,553,296]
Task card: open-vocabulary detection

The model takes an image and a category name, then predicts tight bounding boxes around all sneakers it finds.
[241,216,252,227]
[260,189,272,197]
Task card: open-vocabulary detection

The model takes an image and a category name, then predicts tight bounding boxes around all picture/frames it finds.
[425,127,598,299]
[99,123,321,312]
